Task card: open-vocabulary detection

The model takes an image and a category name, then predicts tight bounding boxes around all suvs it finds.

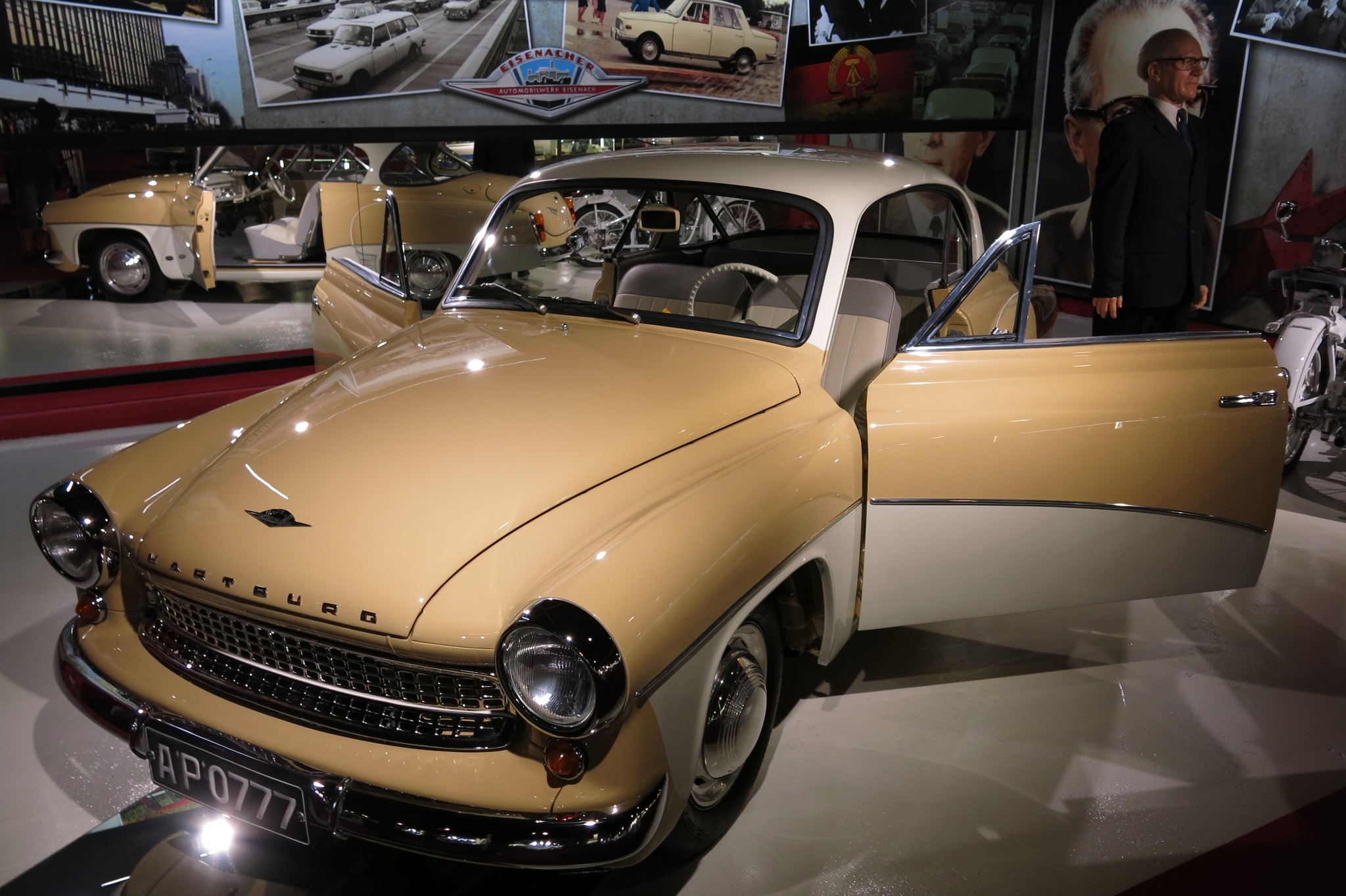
[293,11,427,94]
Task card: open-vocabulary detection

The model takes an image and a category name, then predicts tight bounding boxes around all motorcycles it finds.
[572,186,766,266]
[1264,199,1346,473]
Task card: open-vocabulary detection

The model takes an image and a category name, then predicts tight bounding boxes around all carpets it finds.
[2,345,316,440]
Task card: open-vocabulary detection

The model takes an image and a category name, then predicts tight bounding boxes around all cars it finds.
[270,0,332,22]
[305,2,379,44]
[442,0,479,20]
[31,146,1288,878]
[241,0,262,15]
[611,0,779,75]
[414,0,439,11]
[38,137,578,301]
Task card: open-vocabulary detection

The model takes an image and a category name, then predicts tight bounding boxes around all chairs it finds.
[612,260,765,328]
[749,272,905,436]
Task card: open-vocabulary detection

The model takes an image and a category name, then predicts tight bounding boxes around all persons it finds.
[0,76,220,143]
[1295,0,1345,57]
[254,0,418,65]
[576,0,783,42]
[1086,27,1213,339]
[1031,1,1226,308]
[1246,0,1298,41]
[880,132,1010,260]
[809,0,920,48]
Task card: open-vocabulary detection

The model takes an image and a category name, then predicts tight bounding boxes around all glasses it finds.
[1149,56,1209,72]
[1067,83,1218,127]
